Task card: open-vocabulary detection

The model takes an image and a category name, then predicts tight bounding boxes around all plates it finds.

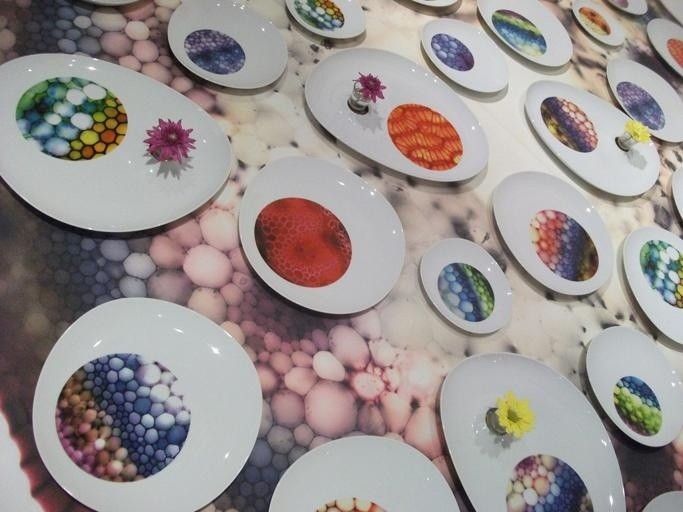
[32,294,266,512]
[575,1,624,46]
[239,154,406,315]
[647,17,682,76]
[286,0,367,40]
[421,19,512,93]
[303,48,491,185]
[439,348,627,511]
[0,50,233,239]
[421,238,512,337]
[416,0,460,8]
[671,166,682,217]
[605,56,682,143]
[492,169,615,297]
[642,490,681,511]
[167,0,290,90]
[610,1,650,15]
[274,436,462,510]
[475,1,574,67]
[523,80,661,197]
[587,325,683,448]
[621,224,682,346]
